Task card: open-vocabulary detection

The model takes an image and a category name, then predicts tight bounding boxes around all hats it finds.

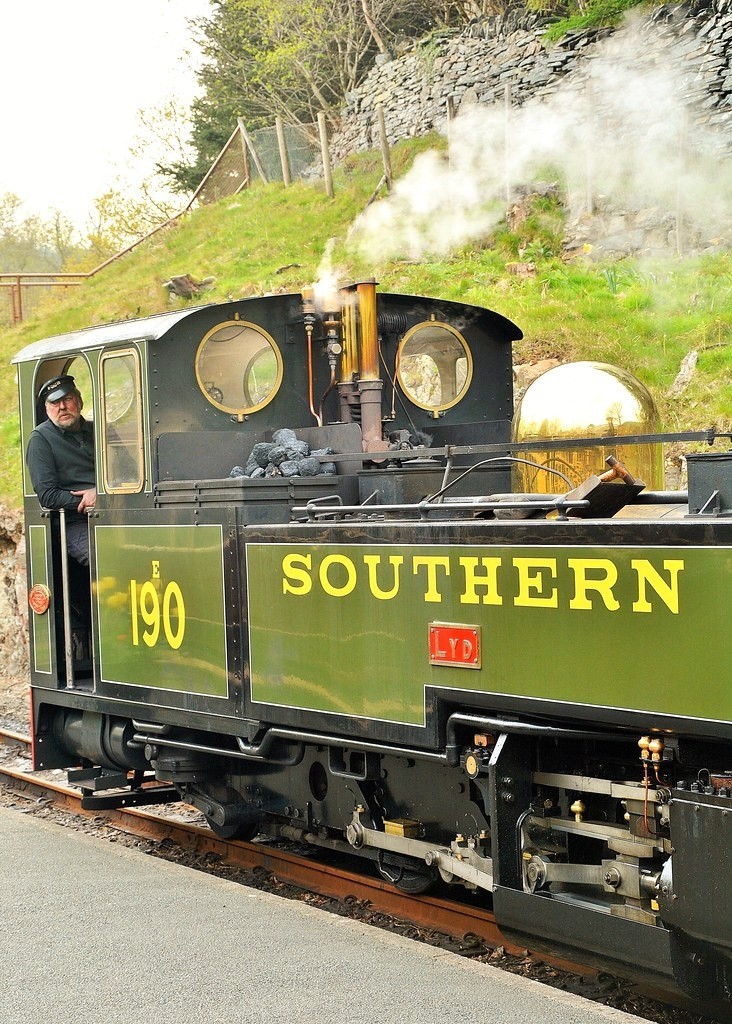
[37,375,75,402]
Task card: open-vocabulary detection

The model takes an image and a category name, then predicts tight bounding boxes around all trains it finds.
[12,273,731,1023]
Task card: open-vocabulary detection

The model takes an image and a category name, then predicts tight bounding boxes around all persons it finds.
[25,374,140,568]
